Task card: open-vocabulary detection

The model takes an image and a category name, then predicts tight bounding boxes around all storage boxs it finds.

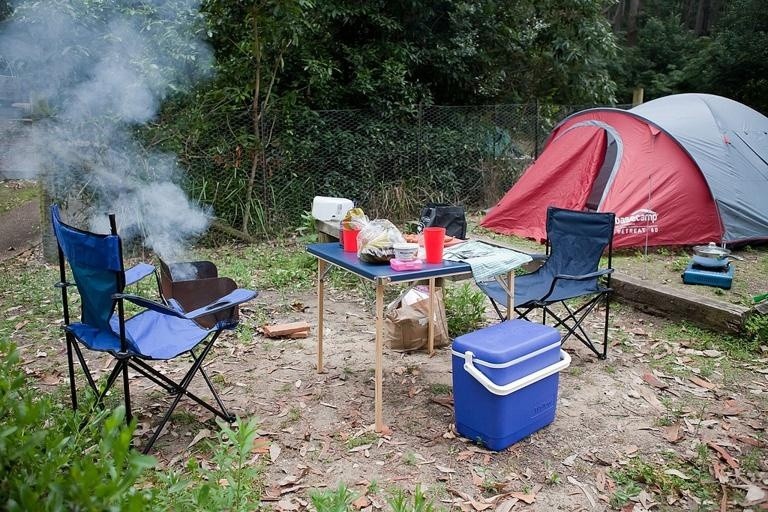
[450,318,571,452]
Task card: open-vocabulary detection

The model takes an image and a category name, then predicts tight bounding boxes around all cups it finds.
[422,227,446,264]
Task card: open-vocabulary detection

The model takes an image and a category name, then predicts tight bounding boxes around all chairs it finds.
[476,206,616,360]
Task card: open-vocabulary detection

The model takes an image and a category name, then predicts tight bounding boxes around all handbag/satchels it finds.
[419,203,467,239]
[387,286,449,353]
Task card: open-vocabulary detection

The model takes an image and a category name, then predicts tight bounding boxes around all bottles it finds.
[339,220,344,248]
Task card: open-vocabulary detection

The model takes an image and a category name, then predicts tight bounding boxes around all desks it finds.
[306,241,535,433]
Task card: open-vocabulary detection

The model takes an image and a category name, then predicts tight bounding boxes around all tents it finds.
[478,93,768,255]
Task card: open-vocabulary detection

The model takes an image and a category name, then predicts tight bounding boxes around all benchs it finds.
[314,220,342,266]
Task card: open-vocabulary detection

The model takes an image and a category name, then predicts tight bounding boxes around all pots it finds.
[692,241,744,268]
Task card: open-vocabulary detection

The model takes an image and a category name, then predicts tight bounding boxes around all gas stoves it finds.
[681,258,735,288]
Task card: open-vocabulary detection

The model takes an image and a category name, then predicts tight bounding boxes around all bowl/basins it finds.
[393,243,418,261]
